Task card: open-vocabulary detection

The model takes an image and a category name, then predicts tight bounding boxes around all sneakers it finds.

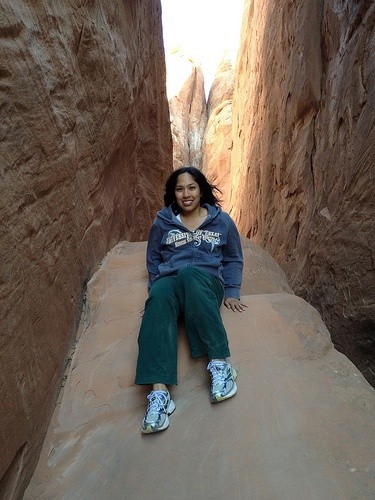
[140,390,176,434]
[206,359,239,404]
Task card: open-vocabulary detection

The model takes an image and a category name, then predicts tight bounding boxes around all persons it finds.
[132,163,248,435]
